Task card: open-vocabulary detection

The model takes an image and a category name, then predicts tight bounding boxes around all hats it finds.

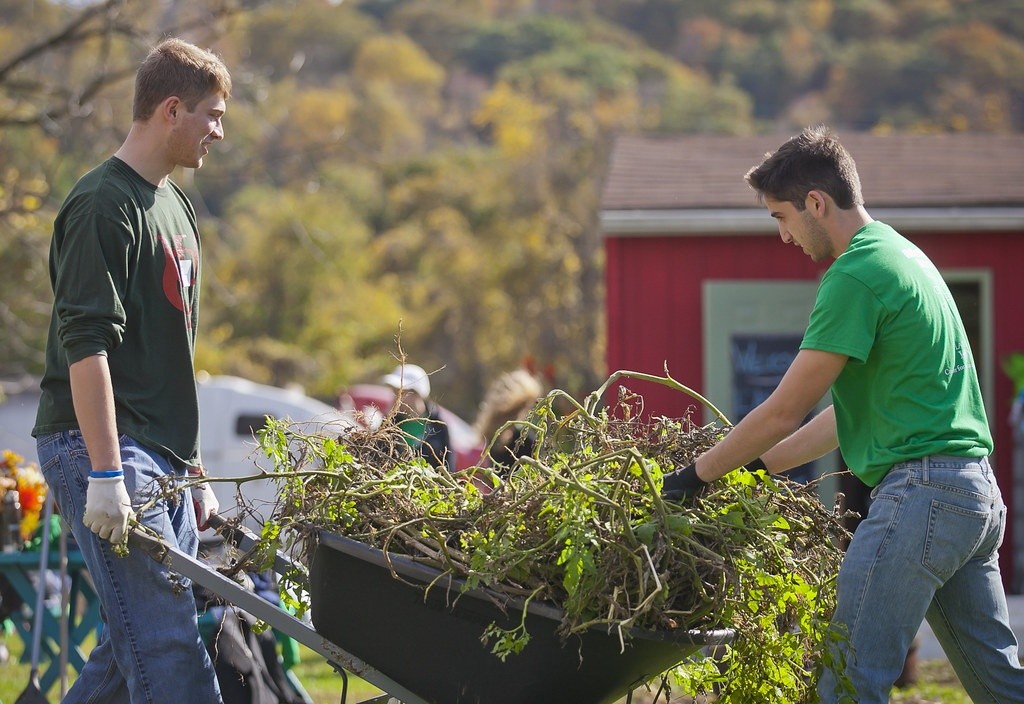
[382,361,431,398]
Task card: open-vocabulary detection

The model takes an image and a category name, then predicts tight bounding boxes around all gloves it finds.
[82,470,136,545]
[186,465,219,532]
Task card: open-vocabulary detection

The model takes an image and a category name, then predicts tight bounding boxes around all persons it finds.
[30,36,232,703]
[381,363,454,478]
[479,371,544,469]
[660,129,1024,704]
